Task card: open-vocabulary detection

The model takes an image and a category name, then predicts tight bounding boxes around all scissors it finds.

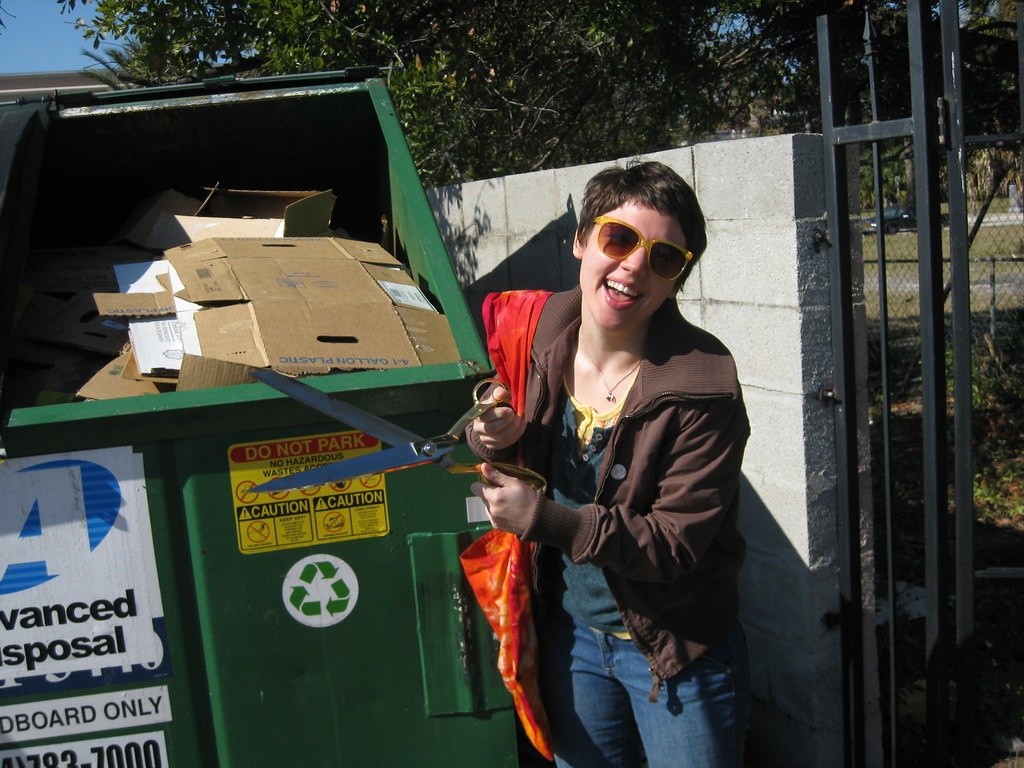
[241,364,548,496]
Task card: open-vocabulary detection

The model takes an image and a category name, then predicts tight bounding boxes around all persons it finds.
[465,153,750,768]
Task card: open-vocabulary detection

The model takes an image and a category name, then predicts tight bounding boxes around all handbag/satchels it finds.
[459,528,552,762]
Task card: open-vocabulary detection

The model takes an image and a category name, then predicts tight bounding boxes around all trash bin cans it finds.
[1,65,520,768]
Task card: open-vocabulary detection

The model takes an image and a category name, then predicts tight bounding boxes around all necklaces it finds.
[580,321,640,404]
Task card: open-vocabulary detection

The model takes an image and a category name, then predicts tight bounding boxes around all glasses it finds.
[594,216,694,281]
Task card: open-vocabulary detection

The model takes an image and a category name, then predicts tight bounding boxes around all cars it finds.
[864,208,950,234]
